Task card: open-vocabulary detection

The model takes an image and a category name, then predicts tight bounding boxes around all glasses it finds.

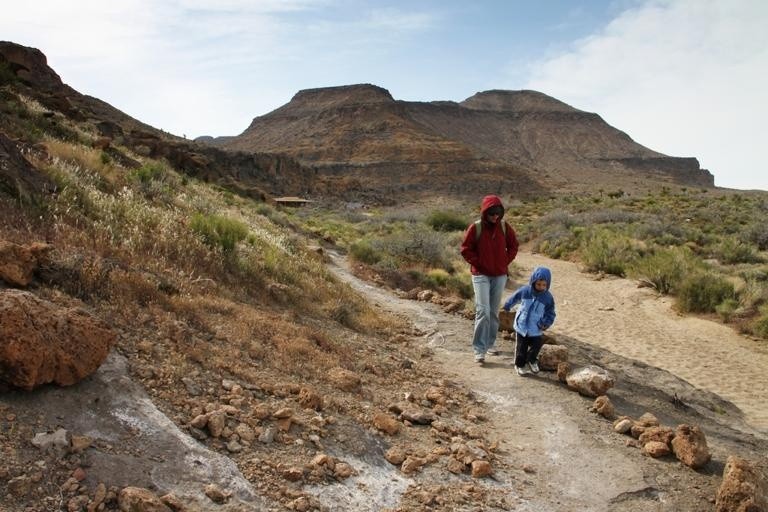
[489,210,501,216]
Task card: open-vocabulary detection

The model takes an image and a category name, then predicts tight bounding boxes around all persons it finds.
[501,267,556,376]
[460,195,519,363]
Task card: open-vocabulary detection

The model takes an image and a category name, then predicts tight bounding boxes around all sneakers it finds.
[476,348,498,361]
[514,361,539,375]
